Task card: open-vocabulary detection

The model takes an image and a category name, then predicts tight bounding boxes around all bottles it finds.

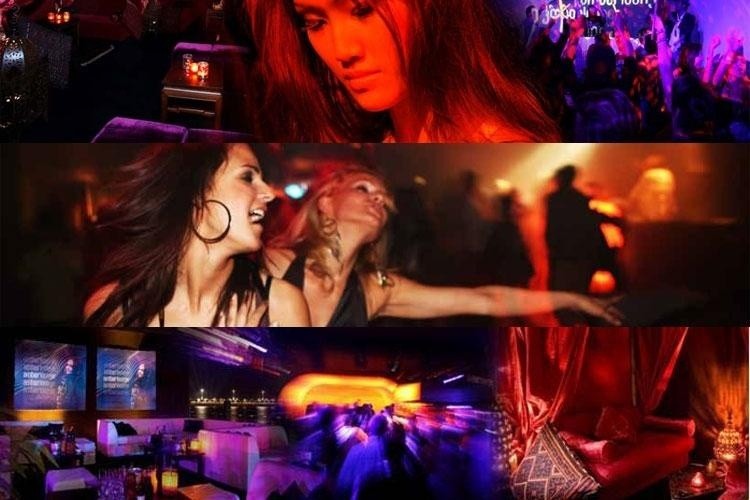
[98,465,157,500]
[49,424,75,456]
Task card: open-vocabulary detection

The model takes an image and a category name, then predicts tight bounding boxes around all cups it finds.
[162,468,179,496]
